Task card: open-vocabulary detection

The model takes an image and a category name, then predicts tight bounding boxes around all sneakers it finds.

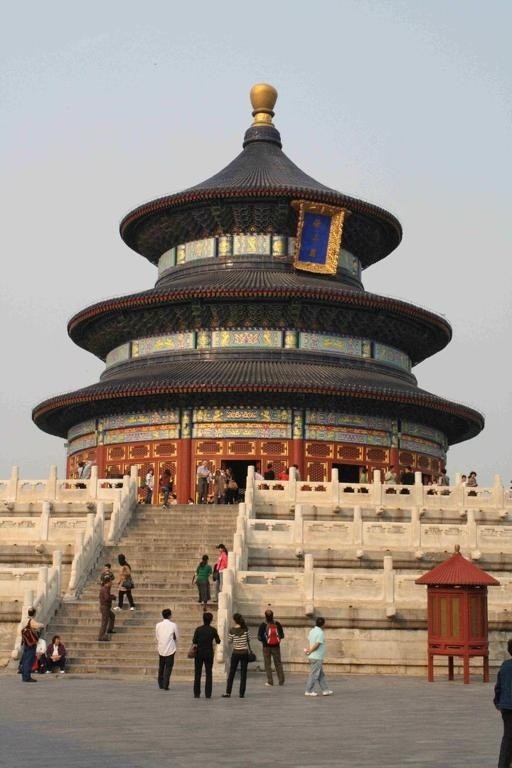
[305,691,318,695]
[265,682,273,686]
[112,606,121,610]
[46,670,52,673]
[130,606,135,610]
[323,689,333,695]
[59,670,64,673]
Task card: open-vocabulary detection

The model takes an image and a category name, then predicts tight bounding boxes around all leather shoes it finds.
[222,693,230,696]
[107,629,116,632]
[99,637,110,640]
[26,678,36,681]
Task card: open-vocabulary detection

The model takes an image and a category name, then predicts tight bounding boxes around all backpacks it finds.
[263,620,280,645]
[21,618,38,646]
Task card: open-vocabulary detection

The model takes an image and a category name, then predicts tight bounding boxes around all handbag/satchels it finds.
[188,643,197,657]
[121,574,134,588]
[37,653,47,673]
[213,571,219,580]
[248,653,256,661]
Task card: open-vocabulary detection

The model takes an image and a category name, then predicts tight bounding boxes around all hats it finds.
[216,543,225,548]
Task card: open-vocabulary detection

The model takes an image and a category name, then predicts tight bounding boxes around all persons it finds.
[195,553,212,610]
[459,469,478,497]
[221,612,253,698]
[17,605,47,682]
[155,607,179,690]
[43,634,69,674]
[491,634,512,766]
[257,608,285,686]
[212,542,229,603]
[192,611,221,698]
[96,576,117,640]
[99,562,115,585]
[70,456,450,509]
[112,551,137,612]
[303,615,335,696]
[33,637,47,673]
